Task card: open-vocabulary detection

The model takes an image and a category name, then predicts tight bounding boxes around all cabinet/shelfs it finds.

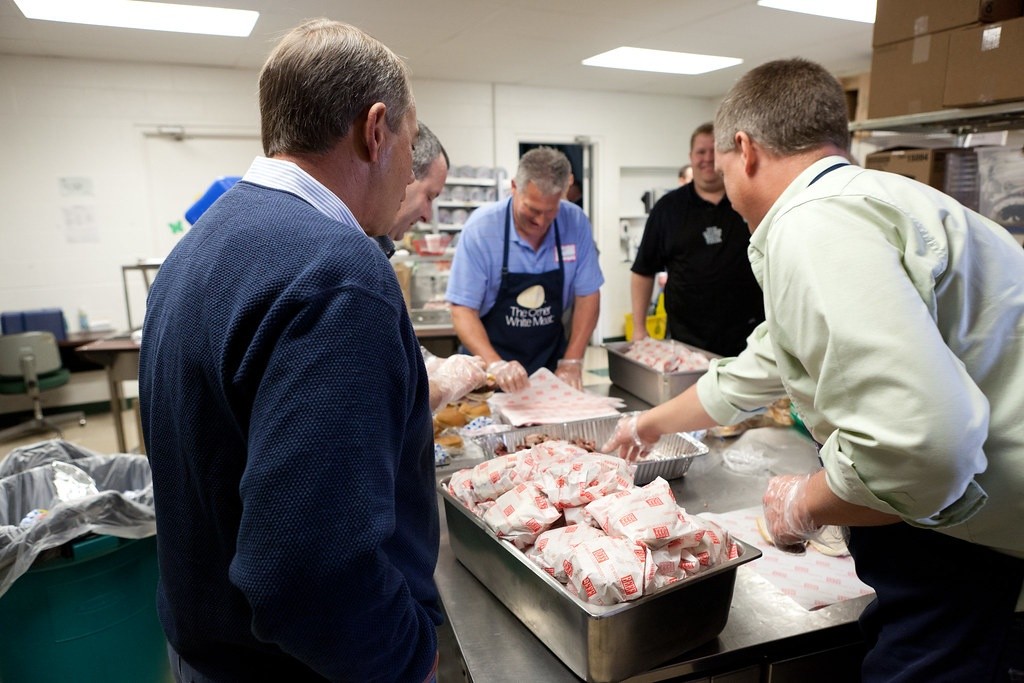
[390,172,506,262]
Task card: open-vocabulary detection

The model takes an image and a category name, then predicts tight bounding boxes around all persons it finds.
[444,150,605,392]
[630,123,766,357]
[602,57,1024,683]
[368,122,486,408]
[138,22,441,682]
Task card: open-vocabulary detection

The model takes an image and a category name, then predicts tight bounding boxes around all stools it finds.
[76,338,143,453]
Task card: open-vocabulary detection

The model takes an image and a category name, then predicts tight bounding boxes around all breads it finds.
[708,399,795,436]
[428,402,490,453]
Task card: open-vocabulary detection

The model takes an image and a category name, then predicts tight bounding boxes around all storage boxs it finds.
[435,469,762,683]
[863,0,1024,191]
[472,410,709,485]
[603,339,724,405]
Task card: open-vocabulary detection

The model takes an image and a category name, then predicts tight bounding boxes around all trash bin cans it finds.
[0,439,172,683]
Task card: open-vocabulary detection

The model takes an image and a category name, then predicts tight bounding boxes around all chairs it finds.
[0,309,87,441]
